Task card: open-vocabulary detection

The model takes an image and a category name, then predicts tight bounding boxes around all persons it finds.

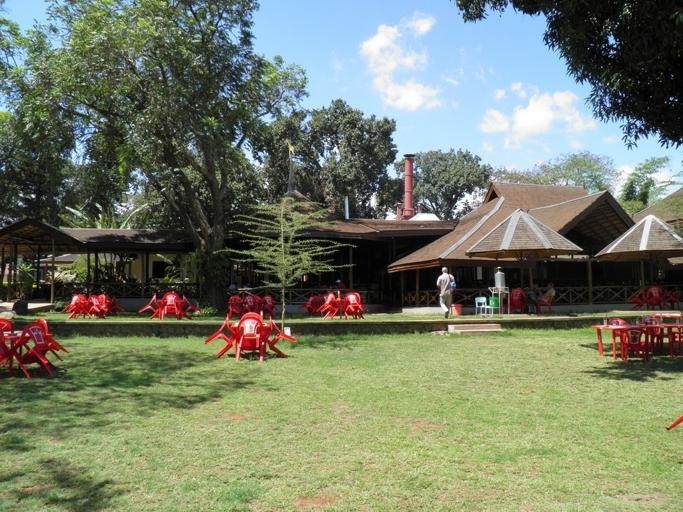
[436,266,456,318]
[334,280,346,289]
[119,274,126,283]
[511,282,556,313]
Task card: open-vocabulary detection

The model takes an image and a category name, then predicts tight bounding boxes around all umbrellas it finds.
[593,214,683,285]
[466,207,585,288]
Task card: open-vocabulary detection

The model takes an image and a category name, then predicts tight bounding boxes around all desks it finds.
[590,324,682,360]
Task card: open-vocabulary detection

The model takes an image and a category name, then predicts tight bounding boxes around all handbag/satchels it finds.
[450,282,457,294]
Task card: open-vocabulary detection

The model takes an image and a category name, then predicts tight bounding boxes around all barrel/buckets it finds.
[451,304,464,316]
[489,297,499,307]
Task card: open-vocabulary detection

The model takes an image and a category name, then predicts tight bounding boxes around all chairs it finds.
[474,296,494,318]
[626,285,680,310]
[0,315,70,380]
[607,313,683,363]
[138,290,369,363]
[61,293,126,319]
